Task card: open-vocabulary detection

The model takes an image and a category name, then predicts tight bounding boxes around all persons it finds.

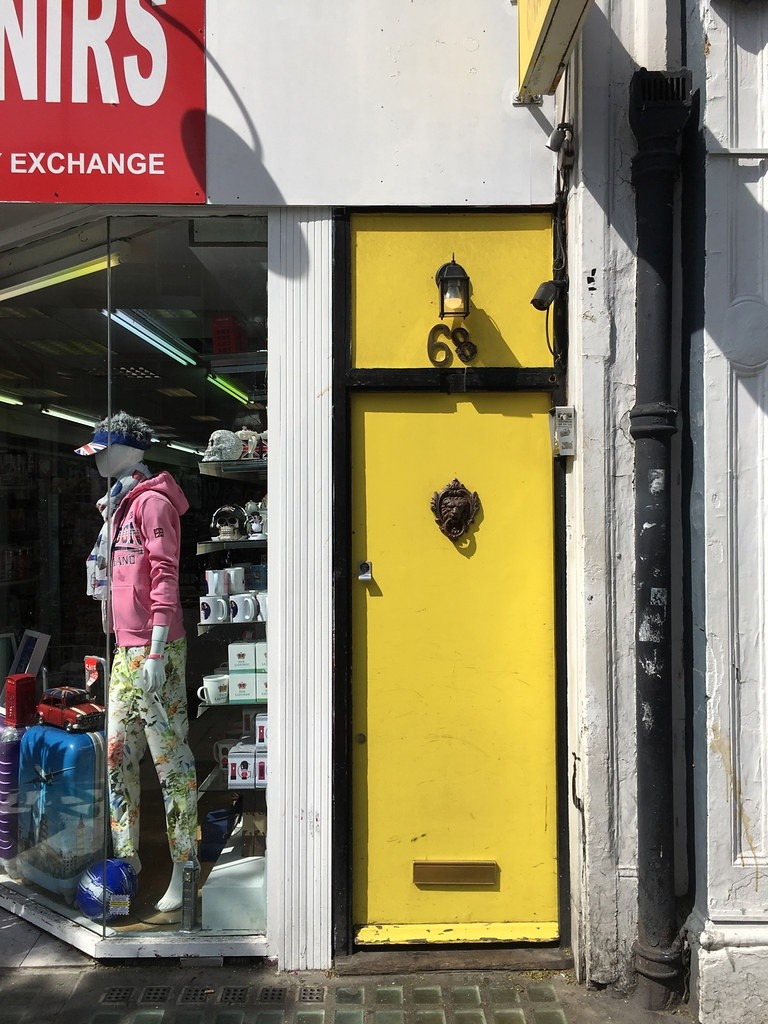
[75,410,200,912]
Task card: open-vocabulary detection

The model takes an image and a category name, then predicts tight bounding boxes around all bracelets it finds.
[146,654,166,661]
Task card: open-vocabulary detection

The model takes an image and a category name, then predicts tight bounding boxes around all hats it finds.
[74,431,151,456]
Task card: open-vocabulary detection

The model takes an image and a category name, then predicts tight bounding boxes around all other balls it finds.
[77,856,141,923]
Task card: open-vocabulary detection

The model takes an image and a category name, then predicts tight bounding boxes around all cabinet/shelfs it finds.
[199,457,268,931]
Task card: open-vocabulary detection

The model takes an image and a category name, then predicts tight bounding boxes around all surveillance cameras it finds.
[530,282,556,310]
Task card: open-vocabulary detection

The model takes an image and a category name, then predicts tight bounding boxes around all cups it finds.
[198,587,268,625]
[234,424,268,462]
[213,738,239,771]
[196,674,229,705]
[213,666,232,676]
[204,565,246,598]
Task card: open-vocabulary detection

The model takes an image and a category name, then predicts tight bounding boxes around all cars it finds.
[34,686,106,734]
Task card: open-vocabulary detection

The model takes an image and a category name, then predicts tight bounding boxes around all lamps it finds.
[435,252,471,320]
[97,308,199,366]
[203,372,255,405]
[40,405,102,428]
[0,241,131,303]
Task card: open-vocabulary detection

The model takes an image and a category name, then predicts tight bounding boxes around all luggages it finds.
[15,725,113,911]
[0,709,37,880]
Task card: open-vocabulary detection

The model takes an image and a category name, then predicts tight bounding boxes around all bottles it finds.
[0,548,28,580]
